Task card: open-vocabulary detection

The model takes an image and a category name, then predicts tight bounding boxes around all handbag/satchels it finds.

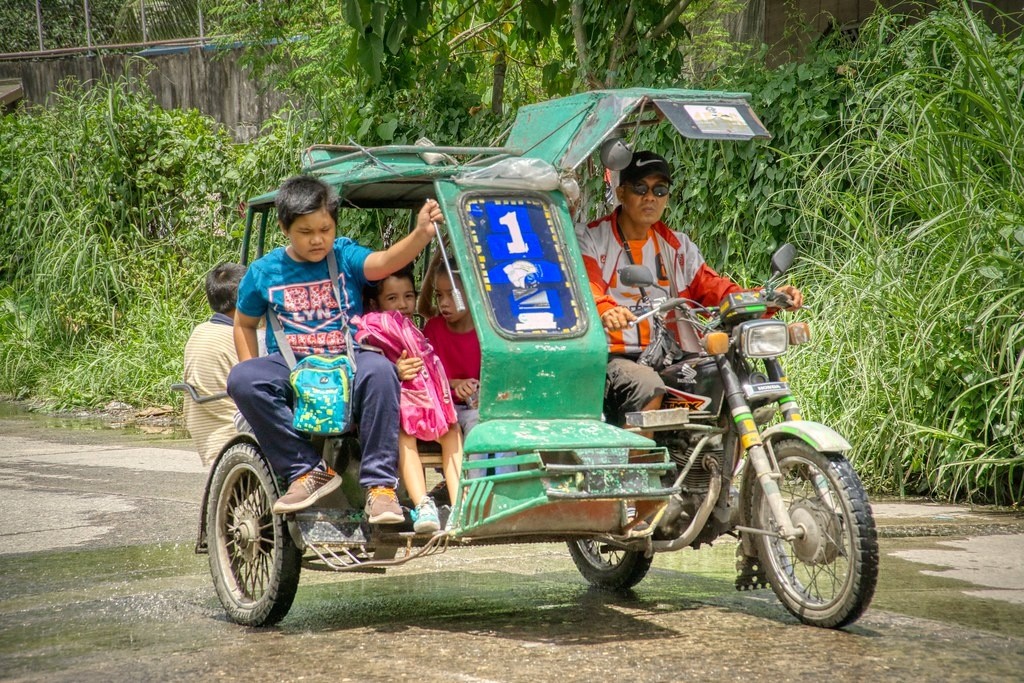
[636,324,685,372]
[289,354,356,436]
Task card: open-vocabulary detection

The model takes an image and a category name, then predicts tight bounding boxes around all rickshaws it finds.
[169,84,880,629]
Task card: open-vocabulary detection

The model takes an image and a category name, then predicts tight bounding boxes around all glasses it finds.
[623,182,670,197]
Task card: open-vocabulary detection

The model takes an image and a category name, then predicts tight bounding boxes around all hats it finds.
[619,150,674,186]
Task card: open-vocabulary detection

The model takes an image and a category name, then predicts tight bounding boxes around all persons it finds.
[559,177,581,232]
[181,261,269,469]
[417,232,519,481]
[227,174,444,525]
[360,262,468,534]
[574,149,804,538]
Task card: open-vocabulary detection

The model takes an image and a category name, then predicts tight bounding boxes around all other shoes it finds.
[629,512,654,536]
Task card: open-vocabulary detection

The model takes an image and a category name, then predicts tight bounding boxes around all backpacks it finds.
[348,310,459,442]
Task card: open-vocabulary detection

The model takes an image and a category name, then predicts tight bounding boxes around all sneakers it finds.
[364,487,405,524]
[410,497,441,533]
[273,458,343,513]
